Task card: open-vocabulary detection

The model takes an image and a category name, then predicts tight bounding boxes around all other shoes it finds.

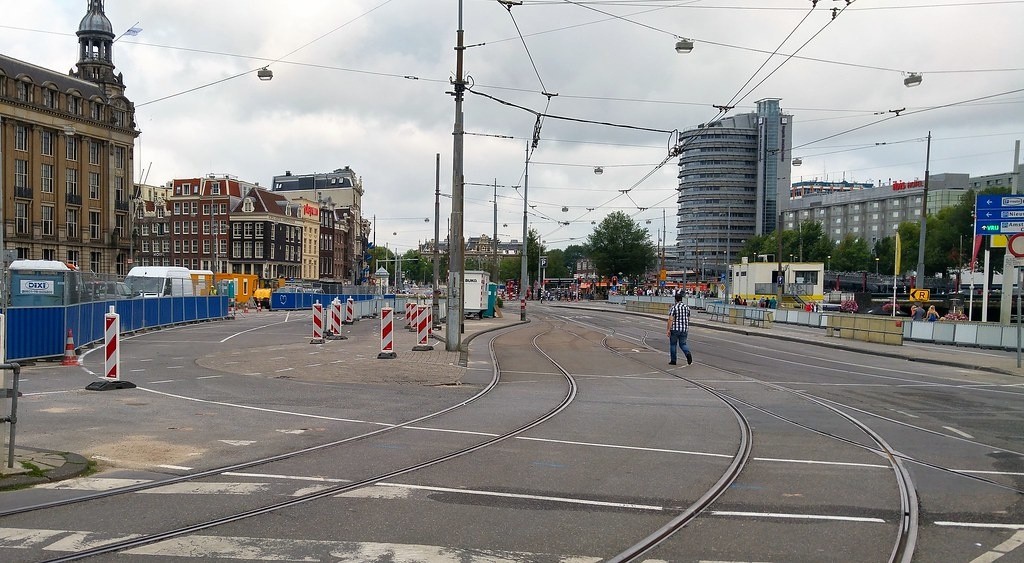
[668,361,676,365]
[686,351,692,365]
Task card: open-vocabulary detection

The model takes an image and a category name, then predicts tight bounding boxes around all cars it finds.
[88,281,133,300]
[269,287,327,305]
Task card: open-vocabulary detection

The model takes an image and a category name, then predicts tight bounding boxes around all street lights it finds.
[754,252,756,263]
[827,255,832,272]
[657,229,663,293]
[790,253,793,262]
[723,250,727,264]
[793,254,798,264]
[876,257,880,277]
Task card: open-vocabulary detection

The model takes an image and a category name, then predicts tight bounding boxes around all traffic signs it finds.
[974,208,1024,220]
[975,195,1024,209]
[975,219,1024,235]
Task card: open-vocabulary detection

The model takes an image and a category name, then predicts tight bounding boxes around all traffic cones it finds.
[257,299,262,312]
[243,302,249,313]
[59,328,80,365]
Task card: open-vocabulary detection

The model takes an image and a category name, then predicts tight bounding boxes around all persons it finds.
[924,305,940,322]
[806,301,818,312]
[911,302,926,322]
[734,295,776,309]
[626,288,706,299]
[666,294,693,365]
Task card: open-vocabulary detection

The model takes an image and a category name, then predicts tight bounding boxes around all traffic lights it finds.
[578,277,581,284]
[613,277,617,286]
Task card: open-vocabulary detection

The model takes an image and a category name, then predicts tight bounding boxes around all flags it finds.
[895,234,901,275]
[970,236,982,270]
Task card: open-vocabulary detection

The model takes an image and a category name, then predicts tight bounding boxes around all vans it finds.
[123,265,194,298]
[7,259,75,304]
